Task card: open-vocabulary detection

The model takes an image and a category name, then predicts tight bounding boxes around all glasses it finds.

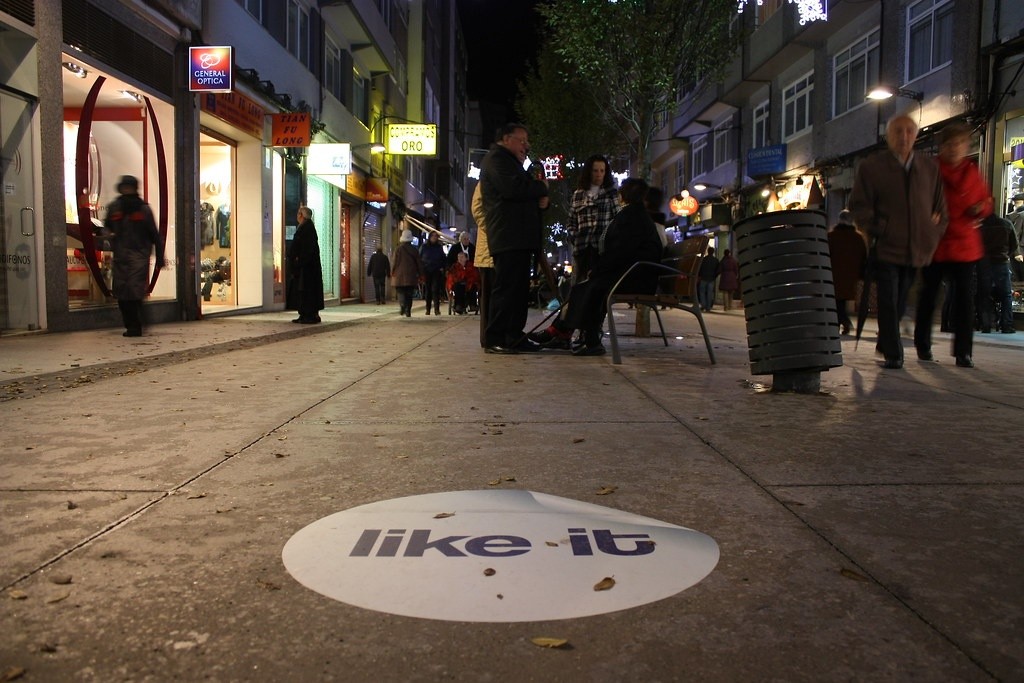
[508,135,529,145]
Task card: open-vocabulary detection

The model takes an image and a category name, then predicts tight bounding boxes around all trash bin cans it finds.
[734,209,846,392]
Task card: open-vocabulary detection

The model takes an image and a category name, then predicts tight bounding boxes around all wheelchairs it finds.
[449,289,480,315]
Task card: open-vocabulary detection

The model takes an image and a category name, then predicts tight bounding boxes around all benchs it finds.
[606,233,719,366]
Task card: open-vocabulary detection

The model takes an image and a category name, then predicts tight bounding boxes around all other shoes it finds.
[884,358,903,369]
[292,317,320,324]
[434,309,441,315]
[841,322,851,335]
[981,328,991,333]
[425,310,430,315]
[405,311,411,317]
[955,354,974,367]
[400,308,406,315]
[123,330,142,337]
[1002,326,1015,333]
[915,347,933,359]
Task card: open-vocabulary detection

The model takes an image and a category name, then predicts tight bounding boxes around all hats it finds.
[116,175,141,193]
[399,230,414,242]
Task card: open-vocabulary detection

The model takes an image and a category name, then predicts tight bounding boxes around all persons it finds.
[527,186,668,350]
[976,193,1024,333]
[529,263,542,308]
[103,176,166,337]
[539,177,664,357]
[846,114,950,369]
[471,180,496,348]
[698,247,722,312]
[392,229,423,317]
[913,123,994,366]
[446,231,475,314]
[418,230,447,316]
[717,249,738,311]
[480,123,551,355]
[288,207,324,324]
[367,247,391,305]
[566,153,626,356]
[827,209,867,336]
[446,252,481,315]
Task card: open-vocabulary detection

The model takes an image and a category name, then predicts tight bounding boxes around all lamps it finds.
[352,142,385,155]
[760,183,786,197]
[119,89,142,102]
[440,226,457,232]
[260,80,275,97]
[244,69,259,86]
[796,173,818,185]
[275,93,292,111]
[62,61,87,79]
[857,86,925,102]
[411,201,434,209]
[693,182,724,194]
[295,100,312,113]
[680,186,690,199]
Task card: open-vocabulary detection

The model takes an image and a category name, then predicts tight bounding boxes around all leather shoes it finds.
[485,343,519,354]
[515,336,543,351]
[540,336,573,350]
[571,343,606,355]
[528,329,551,342]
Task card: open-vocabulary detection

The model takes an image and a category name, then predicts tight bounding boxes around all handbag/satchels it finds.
[452,280,468,299]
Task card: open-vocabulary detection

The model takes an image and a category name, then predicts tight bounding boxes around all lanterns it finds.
[669,194,699,216]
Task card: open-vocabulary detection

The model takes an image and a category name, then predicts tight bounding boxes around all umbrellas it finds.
[854,243,873,351]
